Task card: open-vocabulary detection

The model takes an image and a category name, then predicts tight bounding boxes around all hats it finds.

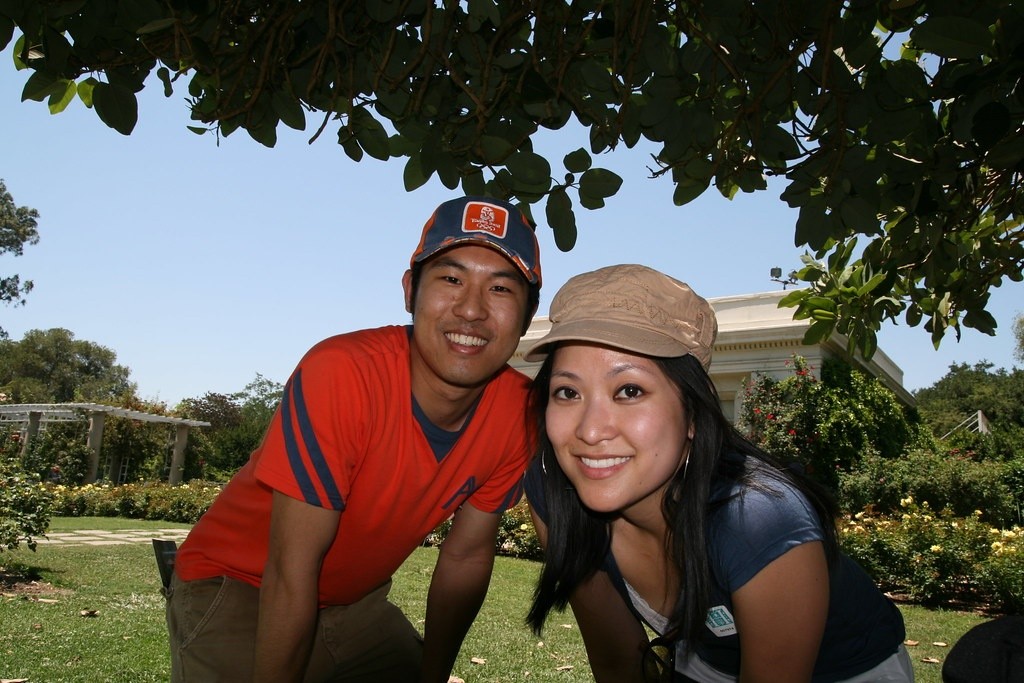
[411,199,544,290]
[523,264,720,373]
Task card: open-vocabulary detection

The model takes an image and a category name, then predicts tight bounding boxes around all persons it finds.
[522,263,916,683]
[163,196,543,683]
